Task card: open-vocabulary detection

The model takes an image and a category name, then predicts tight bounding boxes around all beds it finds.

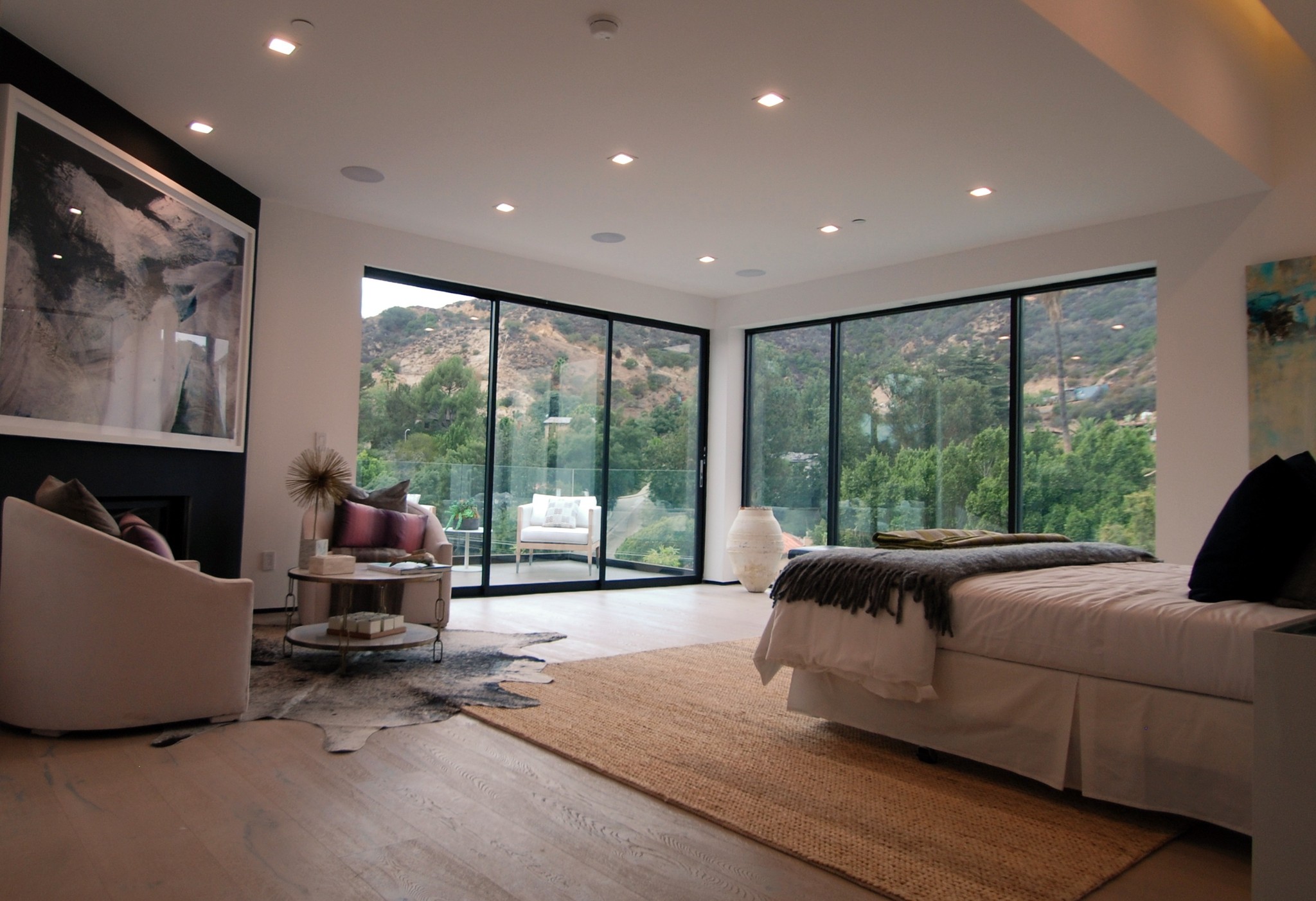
[753,531,1316,837]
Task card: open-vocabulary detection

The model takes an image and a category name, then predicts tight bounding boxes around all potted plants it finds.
[445,500,479,530]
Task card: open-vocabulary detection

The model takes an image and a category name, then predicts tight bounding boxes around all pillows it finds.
[118,512,175,561]
[1188,450,1316,608]
[543,497,581,528]
[34,475,121,538]
[330,498,428,553]
[331,479,411,513]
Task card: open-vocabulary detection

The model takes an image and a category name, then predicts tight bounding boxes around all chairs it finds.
[515,494,602,577]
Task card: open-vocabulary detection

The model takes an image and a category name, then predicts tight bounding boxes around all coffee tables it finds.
[444,526,493,572]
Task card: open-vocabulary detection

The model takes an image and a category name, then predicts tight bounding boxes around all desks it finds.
[280,559,444,672]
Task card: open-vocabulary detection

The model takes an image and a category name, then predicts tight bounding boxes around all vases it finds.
[728,508,783,593]
[298,539,329,568]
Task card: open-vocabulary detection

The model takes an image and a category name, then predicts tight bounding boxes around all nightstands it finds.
[1251,613,1316,901]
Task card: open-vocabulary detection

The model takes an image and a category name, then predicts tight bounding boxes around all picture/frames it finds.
[0,82,255,452]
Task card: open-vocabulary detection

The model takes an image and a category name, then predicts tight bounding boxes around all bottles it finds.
[728,506,784,593]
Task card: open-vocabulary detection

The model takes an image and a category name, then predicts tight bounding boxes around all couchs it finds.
[0,496,254,737]
[300,495,452,629]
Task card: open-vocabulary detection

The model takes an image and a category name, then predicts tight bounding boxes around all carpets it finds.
[459,638,1182,901]
[149,624,566,751]
